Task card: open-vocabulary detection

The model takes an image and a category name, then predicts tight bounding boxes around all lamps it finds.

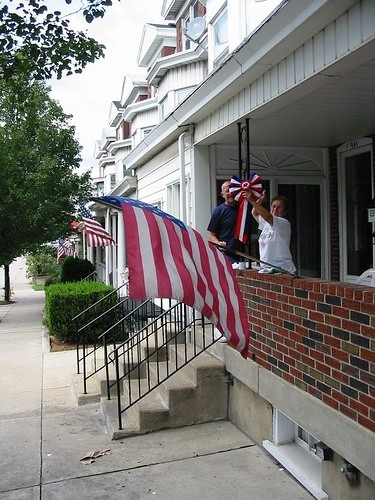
[183,17,208,53]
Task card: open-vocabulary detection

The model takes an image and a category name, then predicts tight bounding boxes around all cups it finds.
[239,261,246,270]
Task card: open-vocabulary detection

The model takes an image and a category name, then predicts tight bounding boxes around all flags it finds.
[56,238,75,263]
[77,203,115,249]
[88,195,251,361]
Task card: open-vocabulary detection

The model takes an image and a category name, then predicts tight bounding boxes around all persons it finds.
[240,189,297,275]
[207,182,254,265]
